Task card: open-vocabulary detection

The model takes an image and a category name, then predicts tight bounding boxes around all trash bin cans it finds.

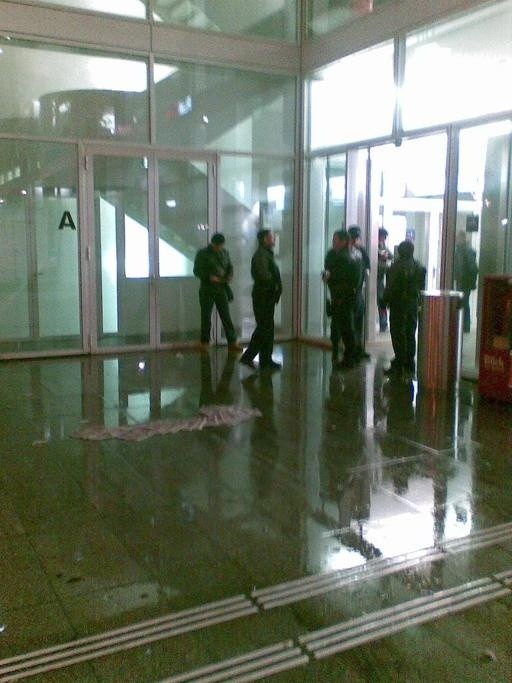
[418,291,459,384]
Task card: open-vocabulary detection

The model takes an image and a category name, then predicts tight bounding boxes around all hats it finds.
[348,227,363,240]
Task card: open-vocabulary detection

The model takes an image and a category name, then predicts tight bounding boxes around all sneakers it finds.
[258,359,282,369]
[239,356,256,371]
[228,339,243,352]
[331,350,417,377]
[192,340,210,356]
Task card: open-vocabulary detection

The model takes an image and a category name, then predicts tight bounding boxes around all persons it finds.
[325,226,372,361]
[383,240,429,375]
[377,225,386,332]
[322,229,360,370]
[193,232,244,355]
[451,227,478,334]
[236,227,281,372]
